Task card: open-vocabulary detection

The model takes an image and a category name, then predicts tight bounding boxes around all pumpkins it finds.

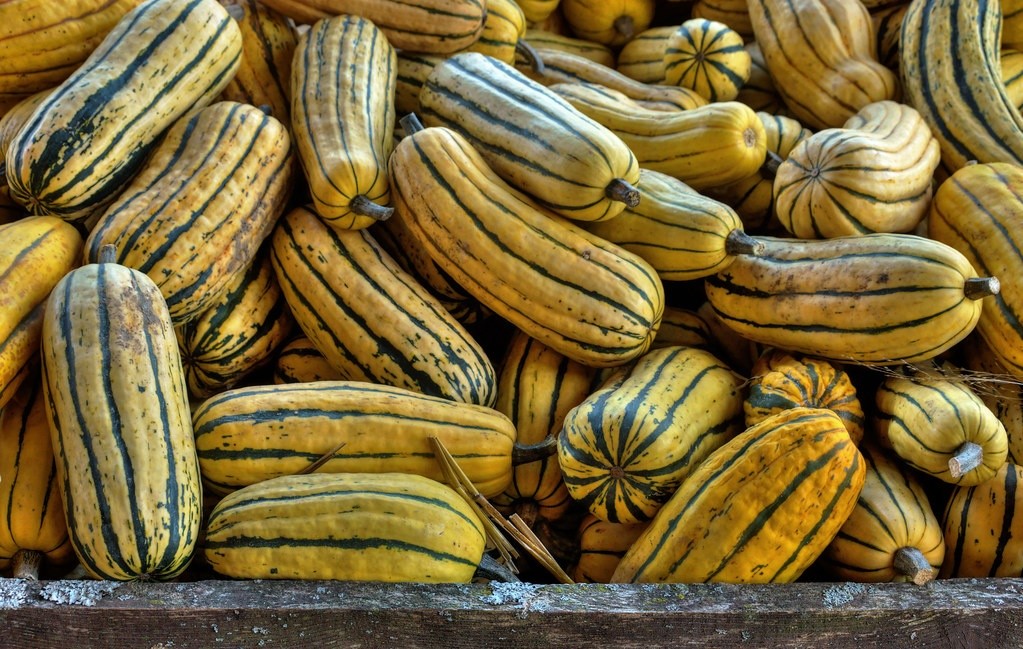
[0,0,1023,585]
